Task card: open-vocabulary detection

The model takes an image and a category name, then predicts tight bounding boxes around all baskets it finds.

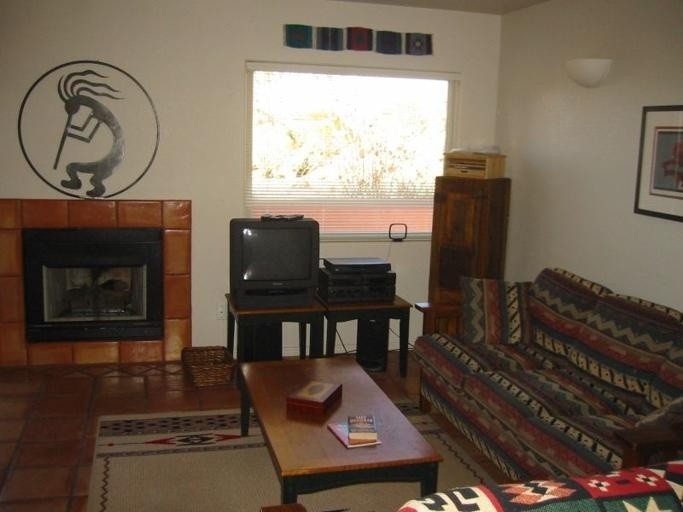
[182,346,235,388]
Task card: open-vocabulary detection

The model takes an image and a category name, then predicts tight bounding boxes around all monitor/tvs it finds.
[229,218,321,309]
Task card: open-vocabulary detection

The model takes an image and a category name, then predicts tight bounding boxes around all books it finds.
[327,415,383,449]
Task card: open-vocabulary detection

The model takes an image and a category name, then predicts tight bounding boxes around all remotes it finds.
[261,212,304,221]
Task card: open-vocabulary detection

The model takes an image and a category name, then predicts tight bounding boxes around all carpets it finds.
[86,398,513,512]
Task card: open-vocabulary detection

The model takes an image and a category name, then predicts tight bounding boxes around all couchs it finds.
[412,268,683,480]
[263,458,683,512]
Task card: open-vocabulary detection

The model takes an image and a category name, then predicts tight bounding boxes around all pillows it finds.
[457,275,532,340]
[635,393,682,427]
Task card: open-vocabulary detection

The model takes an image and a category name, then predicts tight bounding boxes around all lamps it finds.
[566,58,611,87]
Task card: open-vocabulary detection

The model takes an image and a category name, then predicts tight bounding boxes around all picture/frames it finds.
[634,104,683,222]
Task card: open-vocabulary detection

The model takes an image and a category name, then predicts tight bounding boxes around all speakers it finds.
[240,315,283,381]
[356,312,388,372]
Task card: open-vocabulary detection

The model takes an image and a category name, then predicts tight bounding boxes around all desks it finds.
[314,290,413,377]
[225,293,329,438]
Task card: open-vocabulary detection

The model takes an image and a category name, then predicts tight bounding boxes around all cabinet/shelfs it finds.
[423,175,511,334]
